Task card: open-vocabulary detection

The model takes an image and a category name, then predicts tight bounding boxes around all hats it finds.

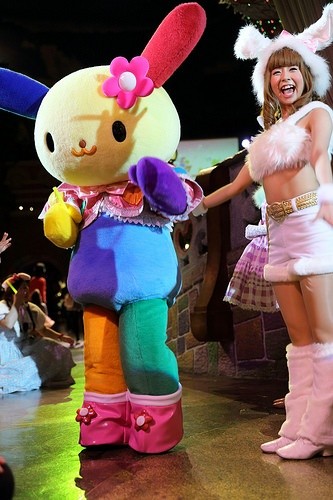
[35,263,46,273]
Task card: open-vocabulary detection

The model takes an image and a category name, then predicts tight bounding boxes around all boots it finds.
[260,344,316,452]
[278,343,333,460]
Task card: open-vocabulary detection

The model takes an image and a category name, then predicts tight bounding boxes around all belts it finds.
[266,191,318,221]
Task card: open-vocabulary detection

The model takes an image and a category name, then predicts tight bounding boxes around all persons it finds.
[192,3,333,460]
[0,232,84,395]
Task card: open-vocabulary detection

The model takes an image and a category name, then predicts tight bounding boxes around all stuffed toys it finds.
[0,4,206,452]
